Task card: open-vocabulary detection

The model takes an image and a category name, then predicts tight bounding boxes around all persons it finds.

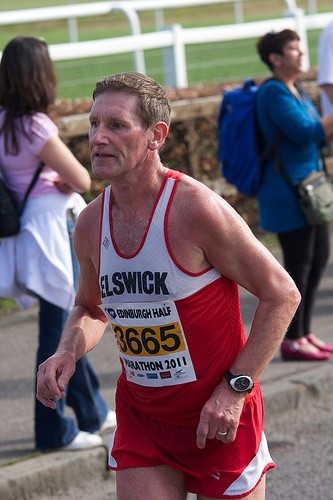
[255,30,333,361]
[316,20,333,153]
[35,72,301,500]
[0,35,117,452]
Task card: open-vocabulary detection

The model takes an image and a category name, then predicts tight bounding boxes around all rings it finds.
[218,430,227,436]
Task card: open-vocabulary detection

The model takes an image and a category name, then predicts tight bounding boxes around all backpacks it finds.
[214,79,300,198]
[0,122,42,238]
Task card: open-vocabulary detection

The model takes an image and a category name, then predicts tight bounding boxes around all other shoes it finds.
[281,334,333,360]
[92,409,116,435]
[59,431,103,451]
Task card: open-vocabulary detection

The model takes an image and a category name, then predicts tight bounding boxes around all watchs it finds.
[223,369,254,393]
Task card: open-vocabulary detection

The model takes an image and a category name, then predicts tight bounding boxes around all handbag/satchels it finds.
[292,170,333,225]
